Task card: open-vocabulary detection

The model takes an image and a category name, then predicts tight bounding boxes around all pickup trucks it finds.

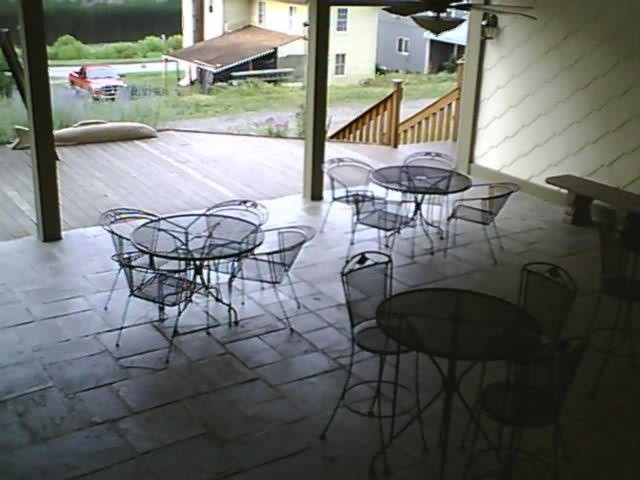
[68,61,129,102]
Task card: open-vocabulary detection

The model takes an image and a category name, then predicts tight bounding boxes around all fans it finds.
[381,1,539,20]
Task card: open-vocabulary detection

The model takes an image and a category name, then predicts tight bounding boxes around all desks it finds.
[366,163,474,256]
[363,285,546,480]
[129,212,267,336]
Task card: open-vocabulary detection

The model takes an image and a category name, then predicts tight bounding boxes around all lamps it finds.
[480,12,501,40]
[411,13,467,37]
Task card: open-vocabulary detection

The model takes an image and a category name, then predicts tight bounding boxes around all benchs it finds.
[546,171,638,225]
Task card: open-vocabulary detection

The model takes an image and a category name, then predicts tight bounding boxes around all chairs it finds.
[512,258,581,336]
[182,198,271,305]
[317,154,391,244]
[98,205,196,323]
[581,204,638,371]
[226,221,316,336]
[317,249,435,458]
[396,150,460,225]
[441,181,523,266]
[107,248,224,371]
[340,187,421,269]
[457,333,588,479]
